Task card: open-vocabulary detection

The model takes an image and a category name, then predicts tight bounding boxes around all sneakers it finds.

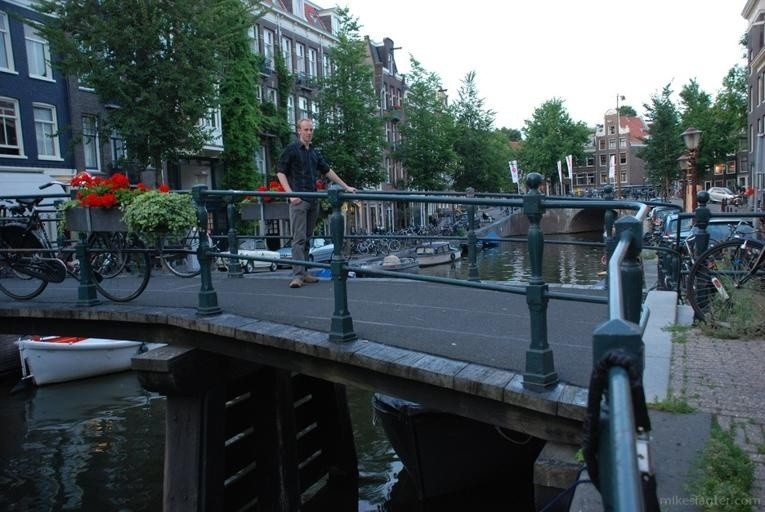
[289,273,322,288]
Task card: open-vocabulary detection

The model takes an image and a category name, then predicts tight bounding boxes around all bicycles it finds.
[688,222,765,334]
[83,220,215,280]
[0,176,154,303]
[349,217,454,256]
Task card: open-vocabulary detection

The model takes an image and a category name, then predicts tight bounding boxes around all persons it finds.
[276,118,358,287]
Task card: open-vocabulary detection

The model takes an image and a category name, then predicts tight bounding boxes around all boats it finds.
[407,241,462,267]
[477,229,500,248]
[459,239,483,253]
[15,368,161,432]
[353,258,422,279]
[15,330,167,386]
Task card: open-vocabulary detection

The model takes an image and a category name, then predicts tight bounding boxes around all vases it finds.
[241,202,290,221]
[64,207,128,233]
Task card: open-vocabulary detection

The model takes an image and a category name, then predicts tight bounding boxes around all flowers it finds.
[245,178,329,203]
[55,170,196,244]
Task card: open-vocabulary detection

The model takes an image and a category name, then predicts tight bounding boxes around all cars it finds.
[276,235,340,271]
[455,212,484,232]
[218,238,281,273]
[589,184,763,297]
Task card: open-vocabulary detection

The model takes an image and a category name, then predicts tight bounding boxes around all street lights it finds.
[680,124,703,217]
[676,153,692,211]
[617,91,626,198]
